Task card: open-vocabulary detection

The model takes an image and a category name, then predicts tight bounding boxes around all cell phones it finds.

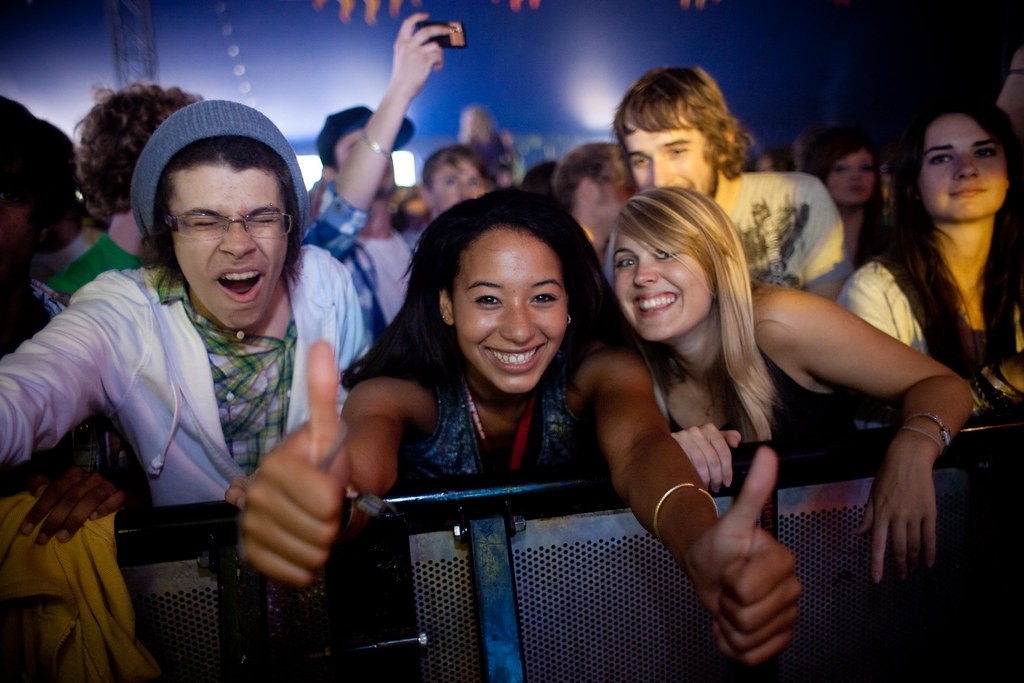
[417,20,468,49]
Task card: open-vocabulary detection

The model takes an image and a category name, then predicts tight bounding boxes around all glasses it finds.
[163,210,294,238]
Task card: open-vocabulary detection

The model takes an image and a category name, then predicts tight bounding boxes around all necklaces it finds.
[684,381,723,417]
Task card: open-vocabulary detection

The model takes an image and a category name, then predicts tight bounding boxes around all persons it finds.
[0,12,974,665]
[995,47,1024,146]
[837,94,1024,465]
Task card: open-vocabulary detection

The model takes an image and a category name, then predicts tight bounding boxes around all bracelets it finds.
[901,425,943,455]
[340,497,354,533]
[906,411,951,446]
[360,129,391,160]
[965,358,1024,417]
[1008,69,1024,75]
[653,483,719,542]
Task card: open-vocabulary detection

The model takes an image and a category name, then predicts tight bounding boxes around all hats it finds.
[130,100,310,249]
[317,107,414,166]
